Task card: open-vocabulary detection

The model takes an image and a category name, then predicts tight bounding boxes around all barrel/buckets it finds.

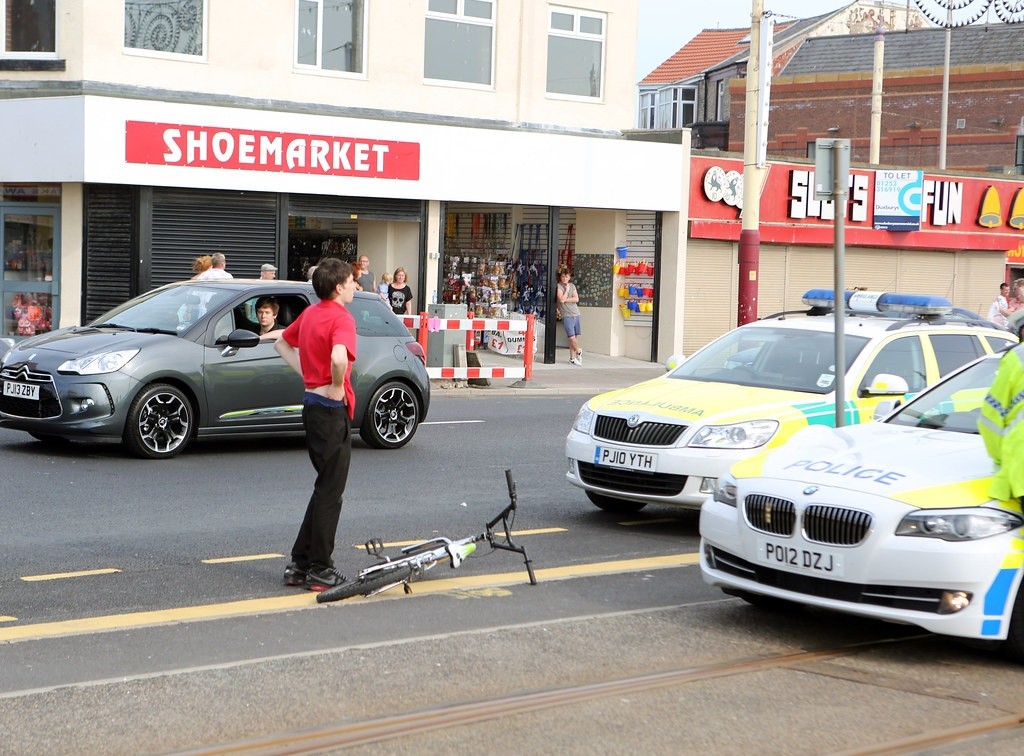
[618,284,654,297]
[619,301,653,318]
[612,260,654,276]
[616,247,627,258]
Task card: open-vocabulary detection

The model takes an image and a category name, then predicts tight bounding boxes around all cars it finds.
[565,289,1024,662]
[0,279,431,460]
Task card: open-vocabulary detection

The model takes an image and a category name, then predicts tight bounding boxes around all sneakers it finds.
[576,349,582,362]
[284,562,306,584]
[569,359,582,366]
[305,568,349,591]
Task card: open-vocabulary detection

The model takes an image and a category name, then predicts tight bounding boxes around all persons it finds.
[557,269,583,365]
[274,258,357,591]
[351,255,377,292]
[184,253,233,322]
[307,266,318,284]
[991,279,1024,331]
[255,297,287,340]
[260,264,278,280]
[977,308,1024,510]
[377,267,413,315]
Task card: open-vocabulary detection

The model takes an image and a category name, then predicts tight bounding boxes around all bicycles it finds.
[315,469,538,604]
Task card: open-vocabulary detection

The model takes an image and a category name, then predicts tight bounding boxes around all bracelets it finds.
[186,311,191,313]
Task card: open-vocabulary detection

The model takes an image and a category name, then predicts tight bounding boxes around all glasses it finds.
[359,260,368,263]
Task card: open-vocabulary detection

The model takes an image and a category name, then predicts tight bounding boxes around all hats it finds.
[261,264,278,271]
[1008,309,1024,335]
